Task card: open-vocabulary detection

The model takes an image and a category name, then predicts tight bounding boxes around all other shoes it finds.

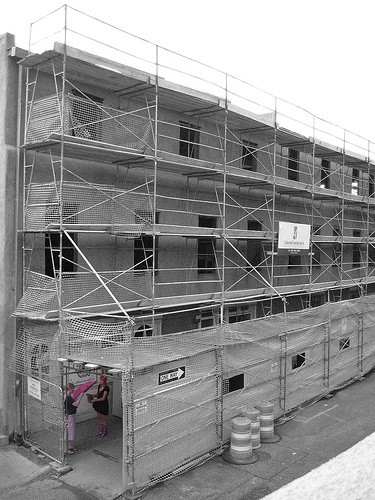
[70,446,79,451]
[68,449,75,456]
[100,432,105,437]
[96,431,102,436]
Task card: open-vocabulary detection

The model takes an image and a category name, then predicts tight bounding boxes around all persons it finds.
[86,375,110,438]
[63,382,84,456]
